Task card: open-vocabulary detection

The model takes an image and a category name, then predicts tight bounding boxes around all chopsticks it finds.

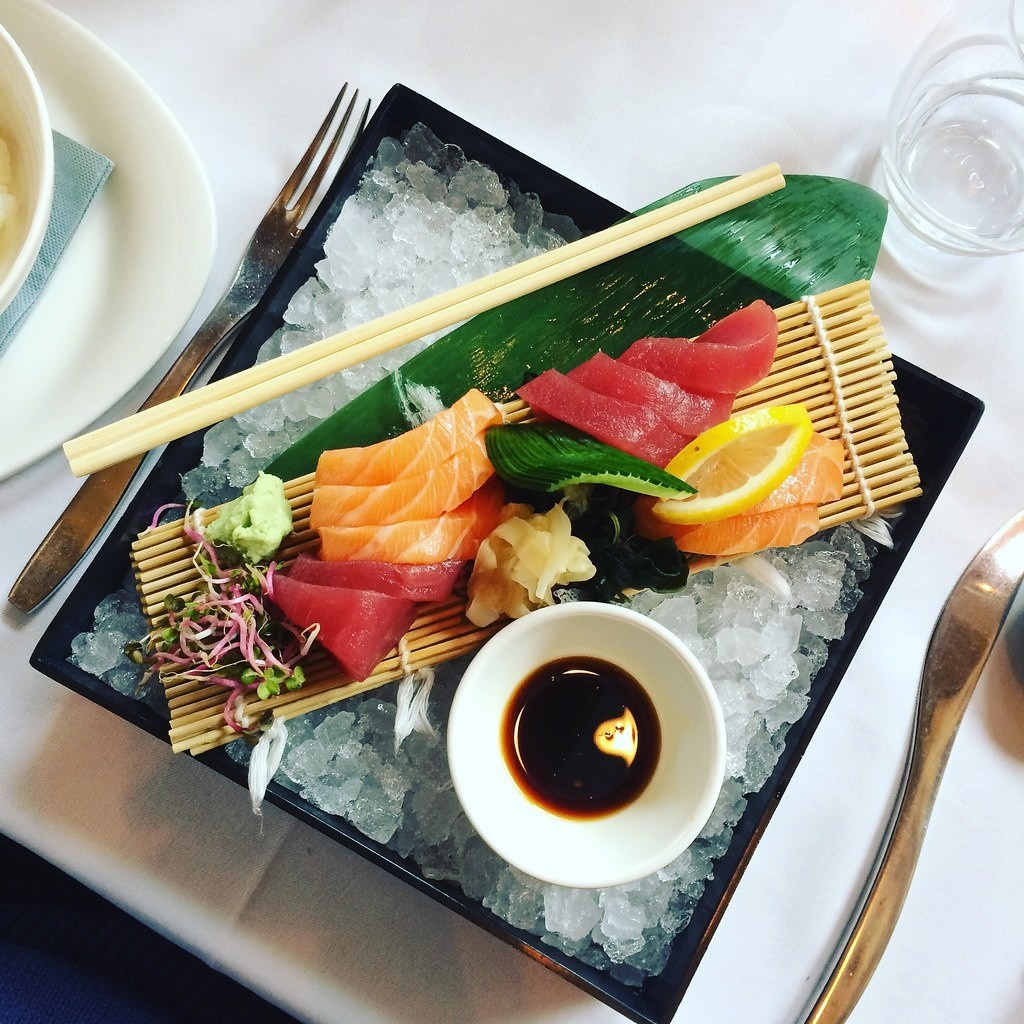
[63,164,786,479]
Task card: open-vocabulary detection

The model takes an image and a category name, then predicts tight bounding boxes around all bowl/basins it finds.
[0,24,55,313]
[446,599,728,889]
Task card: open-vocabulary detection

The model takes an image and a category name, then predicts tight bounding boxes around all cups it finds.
[884,33,1024,254]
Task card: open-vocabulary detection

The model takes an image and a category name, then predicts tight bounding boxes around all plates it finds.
[30,82,984,1024]
[0,1,217,481]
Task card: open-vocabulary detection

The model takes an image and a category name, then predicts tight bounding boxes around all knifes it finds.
[803,506,1023,1024]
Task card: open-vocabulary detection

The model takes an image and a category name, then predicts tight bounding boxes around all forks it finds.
[8,83,373,614]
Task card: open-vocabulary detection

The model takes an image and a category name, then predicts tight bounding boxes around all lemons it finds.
[652,401,811,524]
